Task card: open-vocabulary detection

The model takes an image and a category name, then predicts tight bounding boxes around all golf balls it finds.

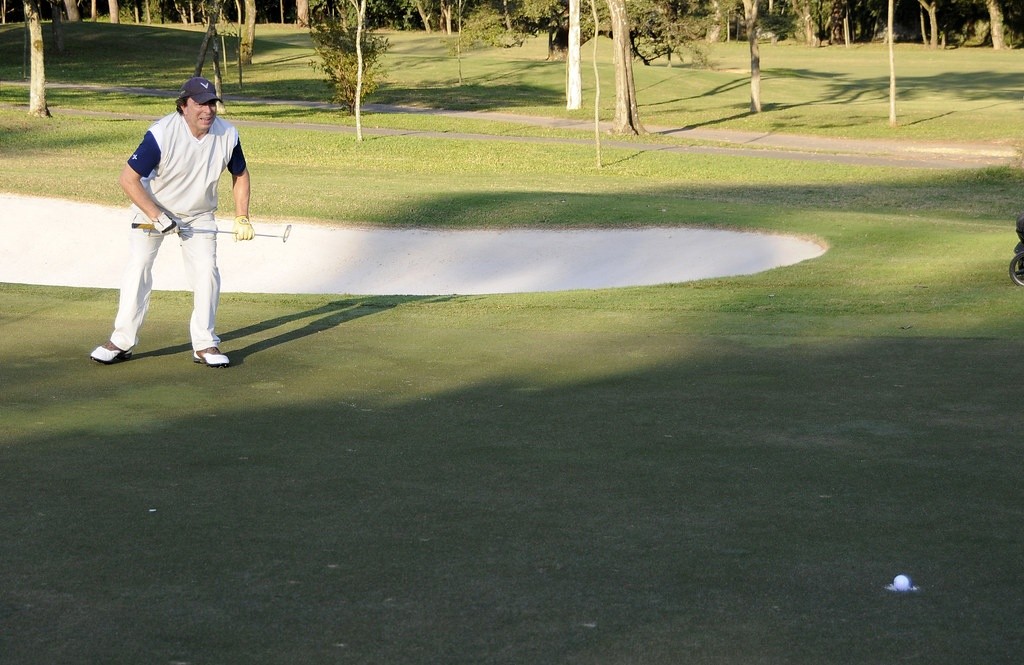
[893,574,909,590]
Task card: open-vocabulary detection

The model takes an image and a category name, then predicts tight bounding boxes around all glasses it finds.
[187,97,217,107]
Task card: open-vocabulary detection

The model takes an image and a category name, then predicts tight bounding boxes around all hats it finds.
[178,77,224,105]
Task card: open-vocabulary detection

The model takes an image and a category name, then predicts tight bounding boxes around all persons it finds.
[87,77,254,368]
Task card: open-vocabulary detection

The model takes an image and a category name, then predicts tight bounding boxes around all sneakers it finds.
[89,341,133,364]
[192,347,230,368]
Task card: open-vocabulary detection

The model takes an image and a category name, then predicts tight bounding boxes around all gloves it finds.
[234,215,255,242]
[151,211,181,235]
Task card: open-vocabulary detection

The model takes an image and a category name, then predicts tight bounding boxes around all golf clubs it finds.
[132,223,292,243]
